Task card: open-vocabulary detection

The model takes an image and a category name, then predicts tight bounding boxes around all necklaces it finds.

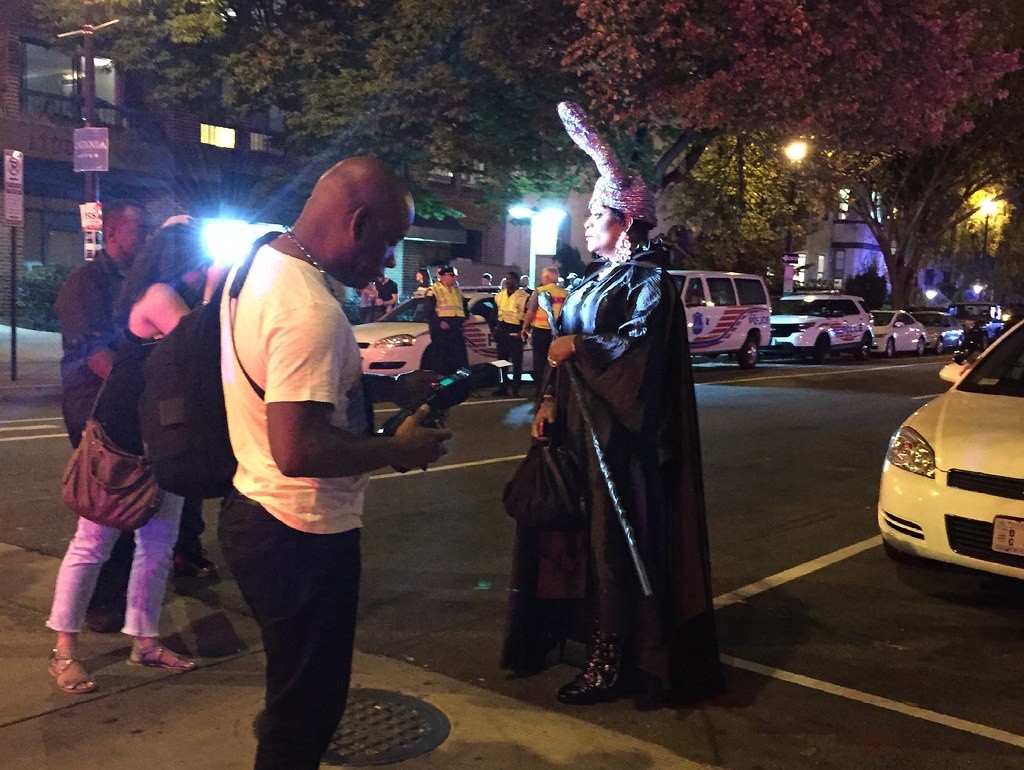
[286,230,337,301]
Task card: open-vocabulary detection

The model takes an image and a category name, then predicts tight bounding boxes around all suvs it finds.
[947,303,1004,351]
[769,295,874,364]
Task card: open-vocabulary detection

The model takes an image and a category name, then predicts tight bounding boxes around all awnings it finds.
[399,207,467,253]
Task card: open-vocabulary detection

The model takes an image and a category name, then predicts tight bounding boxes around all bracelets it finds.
[201,300,212,306]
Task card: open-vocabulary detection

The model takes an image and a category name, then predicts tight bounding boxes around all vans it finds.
[666,270,771,369]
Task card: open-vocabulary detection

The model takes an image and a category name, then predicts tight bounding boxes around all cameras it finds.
[376,359,513,474]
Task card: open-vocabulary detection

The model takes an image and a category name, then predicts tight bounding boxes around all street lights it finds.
[782,142,809,294]
[507,206,567,290]
[980,202,998,257]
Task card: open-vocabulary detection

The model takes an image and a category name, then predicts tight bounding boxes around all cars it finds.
[877,319,1024,580]
[350,287,534,380]
[909,311,965,355]
[870,309,927,358]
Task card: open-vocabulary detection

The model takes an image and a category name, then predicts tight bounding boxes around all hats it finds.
[439,266,459,276]
[558,100,657,226]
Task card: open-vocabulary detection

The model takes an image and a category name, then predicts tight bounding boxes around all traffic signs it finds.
[781,254,799,264]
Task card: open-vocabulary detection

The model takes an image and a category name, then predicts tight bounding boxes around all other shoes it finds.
[514,390,524,398]
[493,389,508,396]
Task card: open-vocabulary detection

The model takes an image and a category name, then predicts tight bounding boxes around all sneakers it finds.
[175,547,215,578]
[86,601,124,633]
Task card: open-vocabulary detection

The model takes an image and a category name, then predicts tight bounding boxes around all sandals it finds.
[125,640,197,671]
[47,651,97,693]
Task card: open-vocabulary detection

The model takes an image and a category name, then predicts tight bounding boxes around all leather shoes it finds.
[556,662,627,706]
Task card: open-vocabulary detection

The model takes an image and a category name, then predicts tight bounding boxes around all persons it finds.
[504,168,720,704]
[217,156,453,770]
[49,198,250,693]
[351,264,580,399]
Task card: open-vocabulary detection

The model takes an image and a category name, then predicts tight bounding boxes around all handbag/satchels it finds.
[502,441,592,532]
[62,418,162,530]
